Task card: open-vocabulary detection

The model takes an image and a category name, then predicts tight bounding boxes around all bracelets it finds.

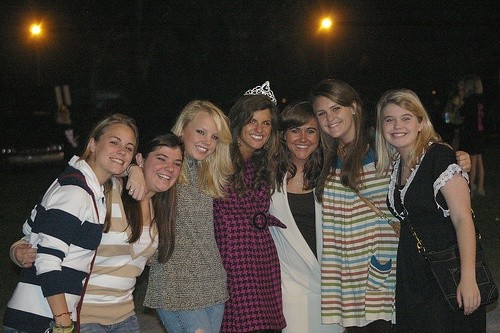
[126,163,136,174]
[53,312,74,333]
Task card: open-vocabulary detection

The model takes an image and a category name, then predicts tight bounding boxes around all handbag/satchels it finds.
[423,235,499,312]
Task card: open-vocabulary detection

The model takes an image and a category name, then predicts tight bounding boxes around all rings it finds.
[129,187,135,192]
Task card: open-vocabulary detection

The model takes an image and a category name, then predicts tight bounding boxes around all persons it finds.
[457,76,486,198]
[308,78,473,333]
[375,88,487,333]
[443,82,467,151]
[0,115,139,333]
[10,134,185,333]
[213,81,288,333]
[268,99,346,333]
[114,100,234,333]
